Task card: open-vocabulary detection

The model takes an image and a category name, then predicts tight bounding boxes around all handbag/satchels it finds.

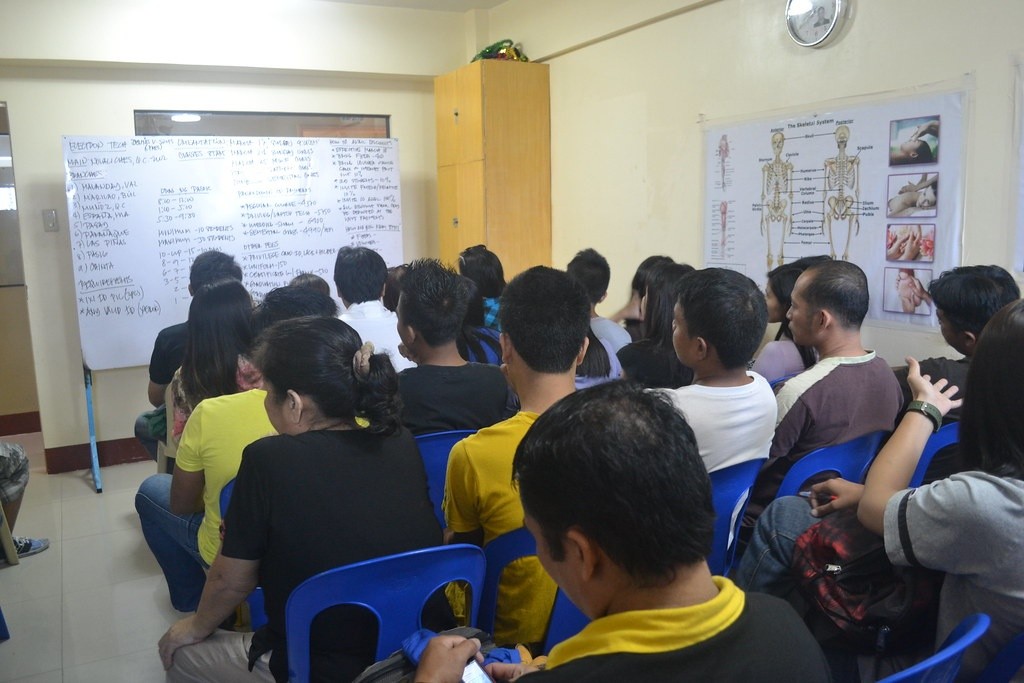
[354,627,496,683]
[792,508,944,657]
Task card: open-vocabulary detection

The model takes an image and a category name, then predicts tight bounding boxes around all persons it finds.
[135,250,264,473]
[736,299,1024,683]
[642,268,777,473]
[134,285,369,631]
[161,315,445,683]
[750,264,820,382]
[738,261,903,552]
[566,249,632,354]
[457,245,508,366]
[417,384,832,683]
[0,438,51,558]
[442,265,592,657]
[615,255,695,387]
[395,258,520,437]
[292,247,418,373]
[892,264,1021,428]
[574,326,622,390]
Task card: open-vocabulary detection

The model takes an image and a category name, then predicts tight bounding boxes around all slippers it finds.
[0,537,49,564]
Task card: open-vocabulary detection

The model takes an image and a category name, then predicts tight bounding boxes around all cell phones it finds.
[461,657,496,683]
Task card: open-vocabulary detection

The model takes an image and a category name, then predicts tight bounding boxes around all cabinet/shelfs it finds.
[435,57,553,283]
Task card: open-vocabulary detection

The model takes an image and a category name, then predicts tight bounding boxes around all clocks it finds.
[785,0,847,48]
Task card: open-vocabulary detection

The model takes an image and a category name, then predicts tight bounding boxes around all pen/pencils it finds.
[799,491,837,501]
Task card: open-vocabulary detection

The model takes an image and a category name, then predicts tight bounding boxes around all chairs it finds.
[219,368,996,683]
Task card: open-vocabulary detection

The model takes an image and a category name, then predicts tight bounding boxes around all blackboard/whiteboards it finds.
[61,134,403,369]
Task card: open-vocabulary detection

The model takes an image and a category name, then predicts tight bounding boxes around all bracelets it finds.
[906,401,942,433]
